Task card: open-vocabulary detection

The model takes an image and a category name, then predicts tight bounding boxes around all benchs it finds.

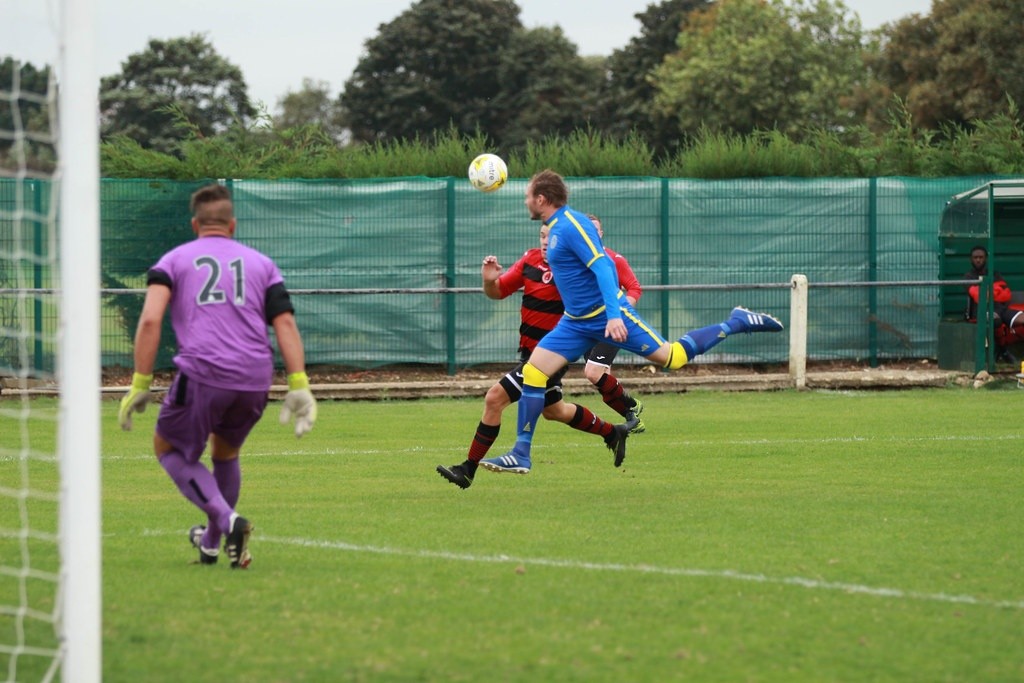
[938,312,1024,373]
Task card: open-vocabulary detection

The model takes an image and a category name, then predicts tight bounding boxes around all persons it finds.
[436,225,629,489]
[584,215,646,434]
[120,185,316,569]
[967,246,1024,361]
[478,169,783,474]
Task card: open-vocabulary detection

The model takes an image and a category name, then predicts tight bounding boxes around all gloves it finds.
[118,374,152,432]
[280,373,318,436]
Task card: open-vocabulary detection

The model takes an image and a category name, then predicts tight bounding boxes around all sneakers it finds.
[731,305,783,332]
[223,518,254,568]
[633,420,644,433]
[436,465,473,490]
[478,451,532,474]
[189,525,217,564]
[630,398,643,417]
[608,424,630,467]
[621,417,640,431]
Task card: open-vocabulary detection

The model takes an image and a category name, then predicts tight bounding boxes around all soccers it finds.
[469,152,508,193]
